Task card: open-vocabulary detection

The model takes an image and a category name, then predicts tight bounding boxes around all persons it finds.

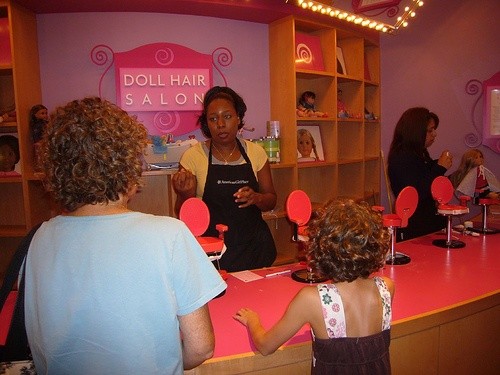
[32,105,49,140]
[388,107,453,242]
[297,130,320,162]
[455,148,500,215]
[0,135,22,177]
[232,201,395,375]
[172,86,276,268]
[16,96,229,375]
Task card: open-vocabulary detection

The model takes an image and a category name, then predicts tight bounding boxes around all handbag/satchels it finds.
[0,224,42,375]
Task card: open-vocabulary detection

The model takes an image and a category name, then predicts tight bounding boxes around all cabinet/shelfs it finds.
[0,0,383,288]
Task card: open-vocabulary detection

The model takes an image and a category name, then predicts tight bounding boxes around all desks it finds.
[183,204,500,375]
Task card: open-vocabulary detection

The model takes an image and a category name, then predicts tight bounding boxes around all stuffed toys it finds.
[297,88,377,119]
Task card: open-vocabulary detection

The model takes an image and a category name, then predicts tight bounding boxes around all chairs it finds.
[468,188,500,234]
[179,197,228,282]
[431,176,469,249]
[380,185,418,265]
[286,190,329,284]
